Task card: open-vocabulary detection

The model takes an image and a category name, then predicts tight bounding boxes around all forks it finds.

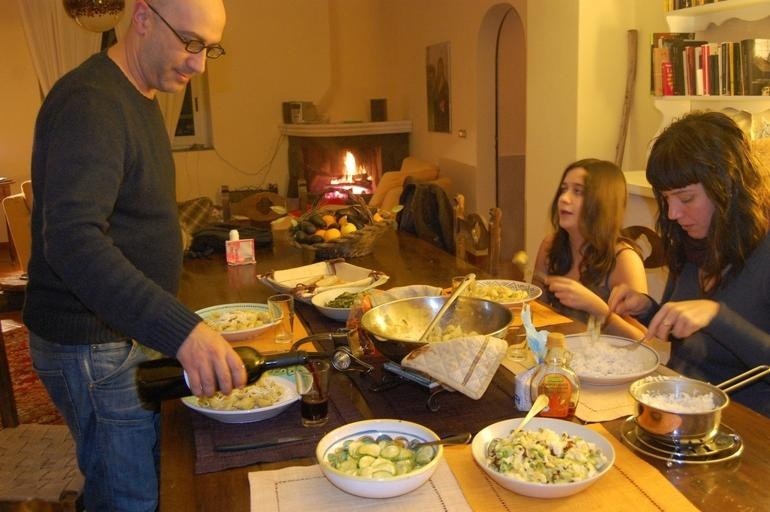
[613,334,648,351]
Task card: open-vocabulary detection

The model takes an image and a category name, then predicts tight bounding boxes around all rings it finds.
[663,320,674,327]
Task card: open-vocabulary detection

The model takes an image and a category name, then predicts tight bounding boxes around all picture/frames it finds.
[424,40,452,136]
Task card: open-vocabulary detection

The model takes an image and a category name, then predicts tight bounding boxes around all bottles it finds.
[135,345,309,407]
[530,332,583,422]
[350,290,388,364]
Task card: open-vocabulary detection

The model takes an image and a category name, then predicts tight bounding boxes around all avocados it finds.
[336,207,367,232]
[296,215,325,243]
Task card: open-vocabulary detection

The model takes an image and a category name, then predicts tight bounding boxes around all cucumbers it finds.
[329,434,435,477]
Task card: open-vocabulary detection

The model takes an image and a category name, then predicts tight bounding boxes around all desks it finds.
[0,178,18,264]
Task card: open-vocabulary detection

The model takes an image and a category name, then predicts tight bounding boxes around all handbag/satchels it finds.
[187,222,273,260]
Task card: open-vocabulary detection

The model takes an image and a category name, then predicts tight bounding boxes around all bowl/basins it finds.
[178,366,314,423]
[474,279,543,307]
[194,302,283,344]
[554,333,661,390]
[314,418,447,500]
[312,288,384,324]
[471,415,618,501]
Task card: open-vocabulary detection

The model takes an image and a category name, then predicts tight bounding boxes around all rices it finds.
[642,377,716,415]
[570,343,646,378]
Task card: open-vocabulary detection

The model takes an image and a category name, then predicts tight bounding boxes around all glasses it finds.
[144,1,225,59]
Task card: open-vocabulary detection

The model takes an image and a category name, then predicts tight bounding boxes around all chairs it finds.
[615,226,669,304]
[450,193,501,275]
[1,331,87,511]
[221,176,309,231]
[400,177,448,250]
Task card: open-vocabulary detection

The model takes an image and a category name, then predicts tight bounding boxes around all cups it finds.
[292,358,328,430]
[370,98,387,122]
[267,294,297,346]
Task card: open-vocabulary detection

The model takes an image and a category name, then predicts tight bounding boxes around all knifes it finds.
[212,432,325,452]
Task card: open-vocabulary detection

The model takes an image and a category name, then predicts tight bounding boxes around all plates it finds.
[255,258,387,307]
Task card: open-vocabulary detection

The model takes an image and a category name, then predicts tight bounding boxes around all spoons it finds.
[485,394,550,459]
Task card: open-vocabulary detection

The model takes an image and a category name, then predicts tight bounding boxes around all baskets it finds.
[286,188,396,260]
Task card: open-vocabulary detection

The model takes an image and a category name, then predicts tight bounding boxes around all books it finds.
[650,34,770,95]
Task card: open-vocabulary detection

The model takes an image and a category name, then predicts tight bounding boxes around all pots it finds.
[356,294,516,365]
[626,363,769,442]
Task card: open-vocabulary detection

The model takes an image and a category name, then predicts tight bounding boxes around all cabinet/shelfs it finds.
[624,0,769,306]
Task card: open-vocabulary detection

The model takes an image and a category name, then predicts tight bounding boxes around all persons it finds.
[608,112,770,419]
[435,58,449,132]
[531,158,649,345]
[22,0,247,512]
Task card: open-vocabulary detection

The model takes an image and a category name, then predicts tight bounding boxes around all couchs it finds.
[367,154,453,219]
[3,181,37,273]
